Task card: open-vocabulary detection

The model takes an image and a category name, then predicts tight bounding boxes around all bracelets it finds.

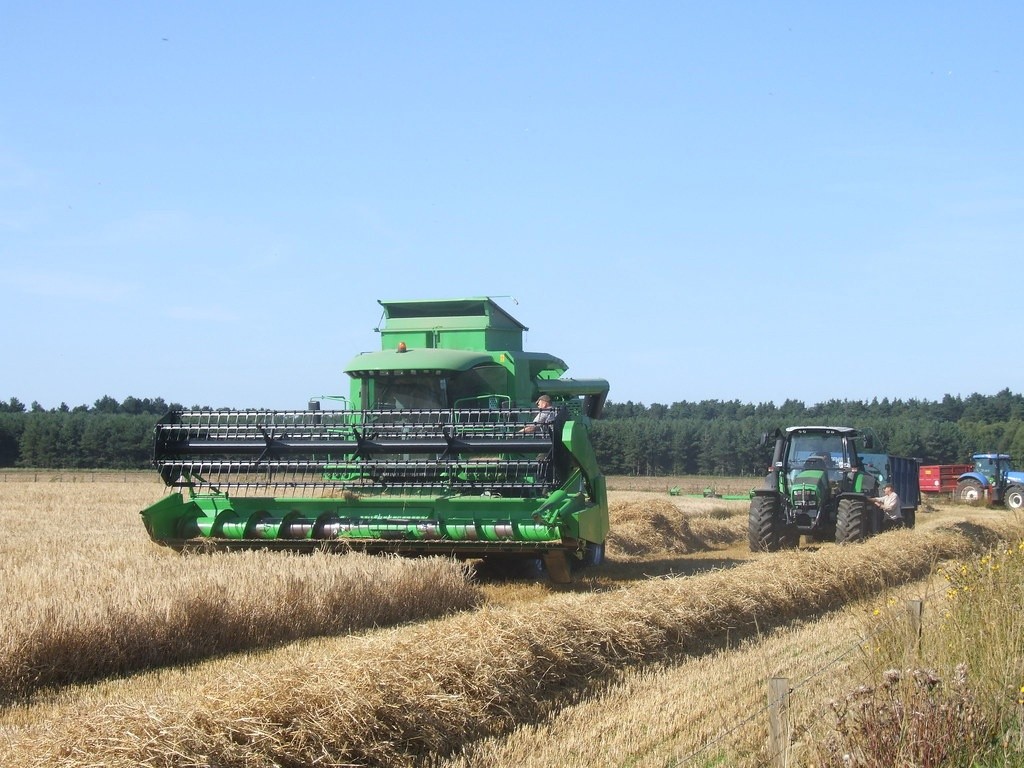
[878,505,881,508]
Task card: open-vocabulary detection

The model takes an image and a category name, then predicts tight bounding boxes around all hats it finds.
[880,483,895,489]
[534,394,551,405]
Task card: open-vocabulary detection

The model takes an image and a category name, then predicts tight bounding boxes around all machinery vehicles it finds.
[918,453,1024,511]
[138,295,611,584]
[748,426,922,553]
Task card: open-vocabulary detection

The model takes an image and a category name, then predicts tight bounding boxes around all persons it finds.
[989,475,996,486]
[870,483,902,532]
[517,395,555,432]
